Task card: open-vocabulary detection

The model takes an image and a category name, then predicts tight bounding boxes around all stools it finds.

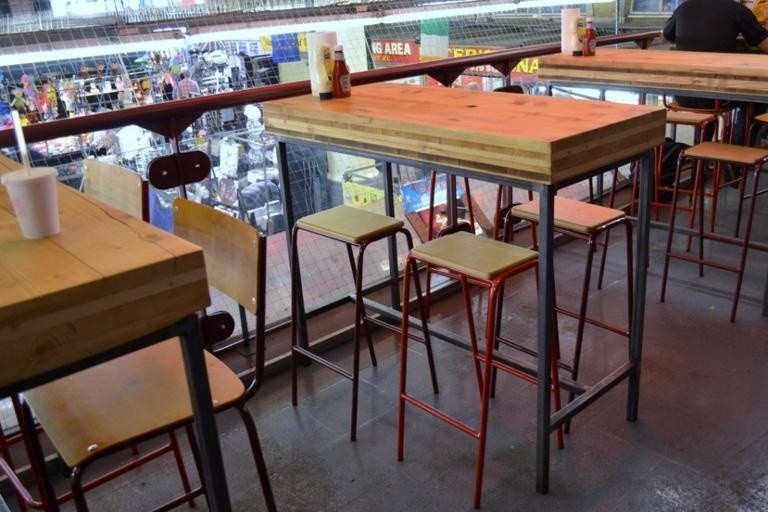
[396,230,567,508]
[286,202,443,445]
[488,194,635,432]
[623,109,718,254]
[656,140,766,323]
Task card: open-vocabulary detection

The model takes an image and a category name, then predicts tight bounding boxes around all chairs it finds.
[18,196,279,512]
[662,44,749,202]
[76,155,157,224]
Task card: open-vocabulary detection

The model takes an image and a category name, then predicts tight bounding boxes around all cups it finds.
[1,165,63,241]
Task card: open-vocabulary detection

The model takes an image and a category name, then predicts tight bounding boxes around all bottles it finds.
[331,43,352,98]
[584,17,597,57]
[311,41,335,100]
[571,14,584,57]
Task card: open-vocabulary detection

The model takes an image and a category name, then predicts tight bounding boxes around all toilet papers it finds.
[559,7,581,56]
[305,30,338,98]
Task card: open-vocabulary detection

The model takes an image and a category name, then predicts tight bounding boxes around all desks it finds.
[538,45,768,272]
[0,149,233,512]
[258,81,670,495]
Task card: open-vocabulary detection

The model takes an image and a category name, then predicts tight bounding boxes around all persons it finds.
[737,1,768,37]
[80,51,255,157]
[661,0,767,190]
